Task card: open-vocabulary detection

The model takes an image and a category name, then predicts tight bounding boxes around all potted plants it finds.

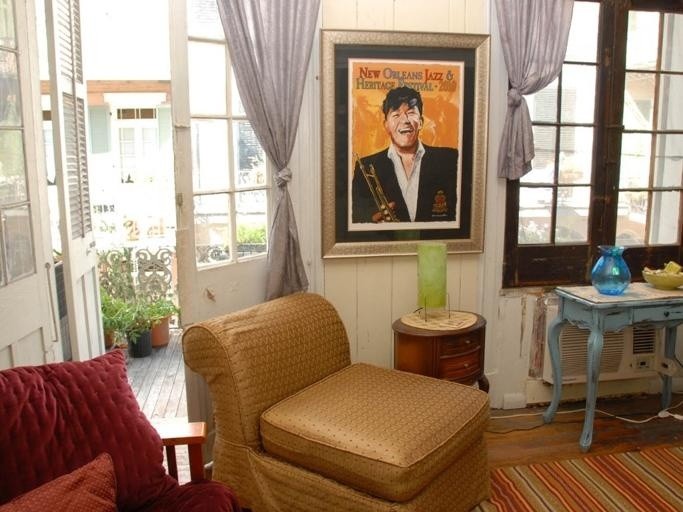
[101,287,179,357]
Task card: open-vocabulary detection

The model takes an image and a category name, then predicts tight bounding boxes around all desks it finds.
[544,280,682,451]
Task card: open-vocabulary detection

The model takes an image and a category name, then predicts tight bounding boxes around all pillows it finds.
[1,347,172,512]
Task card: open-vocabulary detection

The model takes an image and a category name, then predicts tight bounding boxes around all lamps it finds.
[412,241,452,321]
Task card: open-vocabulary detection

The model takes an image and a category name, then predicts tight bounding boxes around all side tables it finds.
[392,306,490,395]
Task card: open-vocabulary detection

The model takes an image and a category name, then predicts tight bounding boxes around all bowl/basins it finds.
[642,270,683,291]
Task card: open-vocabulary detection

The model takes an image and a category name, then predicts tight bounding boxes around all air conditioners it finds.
[536,301,667,384]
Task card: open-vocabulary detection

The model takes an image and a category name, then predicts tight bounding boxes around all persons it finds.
[352,84,454,223]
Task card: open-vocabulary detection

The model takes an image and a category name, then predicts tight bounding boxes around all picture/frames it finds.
[320,27,491,259]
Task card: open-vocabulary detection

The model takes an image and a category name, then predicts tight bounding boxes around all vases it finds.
[590,240,634,296]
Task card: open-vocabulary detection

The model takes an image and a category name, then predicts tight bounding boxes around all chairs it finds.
[182,289,492,512]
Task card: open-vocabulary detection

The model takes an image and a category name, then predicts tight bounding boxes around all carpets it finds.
[480,449,682,511]
